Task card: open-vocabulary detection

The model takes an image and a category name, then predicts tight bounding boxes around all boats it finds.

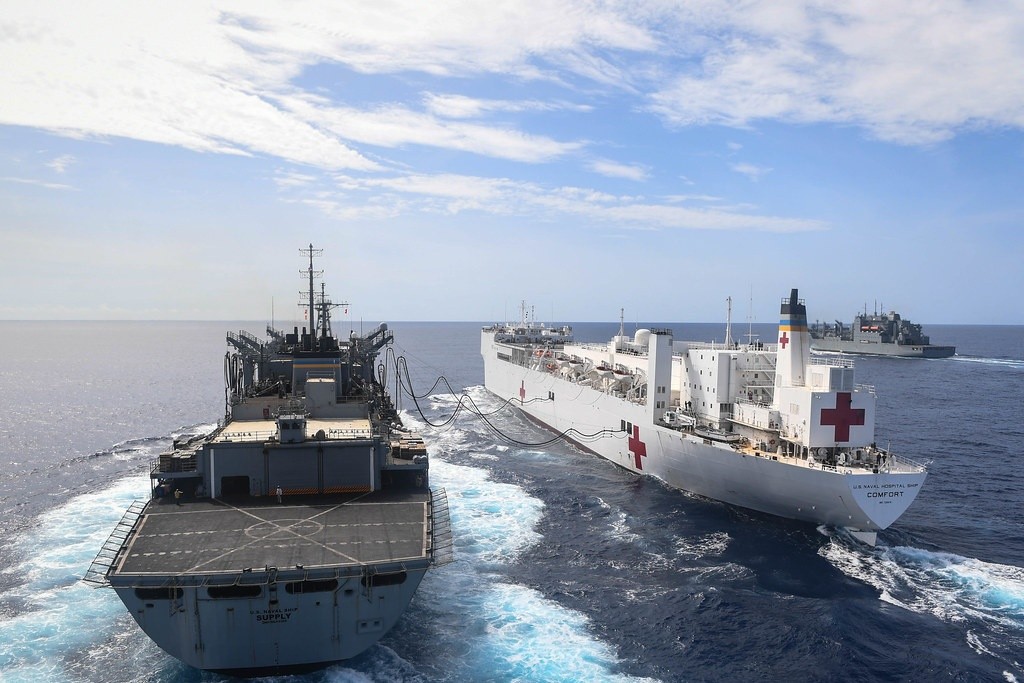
[612,370,631,382]
[568,359,583,369]
[693,424,741,443]
[536,349,550,356]
[555,358,568,366]
[595,366,614,377]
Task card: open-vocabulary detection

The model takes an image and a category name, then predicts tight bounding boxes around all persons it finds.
[175,489,183,505]
[869,441,886,474]
[276,485,283,504]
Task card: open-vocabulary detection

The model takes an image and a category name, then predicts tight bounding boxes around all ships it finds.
[808,299,956,358]
[79,242,453,672]
[478,286,928,529]
